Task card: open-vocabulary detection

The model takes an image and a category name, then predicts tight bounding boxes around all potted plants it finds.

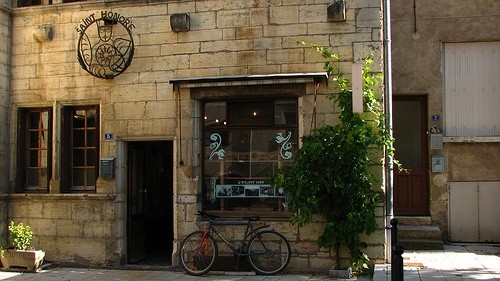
[0,221,45,272]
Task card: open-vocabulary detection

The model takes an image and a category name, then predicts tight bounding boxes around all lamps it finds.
[327,2,346,22]
[36,26,52,40]
[169,13,191,31]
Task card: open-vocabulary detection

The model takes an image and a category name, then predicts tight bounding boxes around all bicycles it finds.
[179,209,291,277]
